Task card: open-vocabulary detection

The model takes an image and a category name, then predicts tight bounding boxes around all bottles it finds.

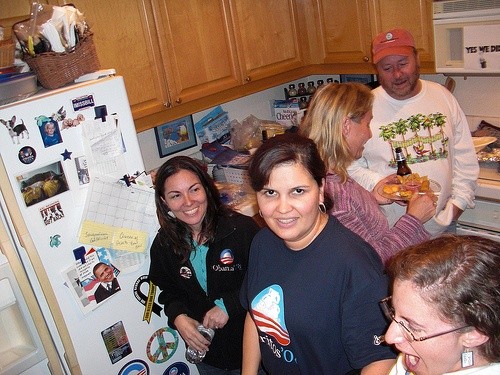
[395,147,412,177]
[298,96,309,110]
[287,77,333,97]
[185,324,215,364]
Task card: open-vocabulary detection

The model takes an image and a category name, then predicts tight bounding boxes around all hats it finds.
[372,29,415,65]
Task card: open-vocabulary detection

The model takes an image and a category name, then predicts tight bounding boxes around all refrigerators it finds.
[0,73,199,375]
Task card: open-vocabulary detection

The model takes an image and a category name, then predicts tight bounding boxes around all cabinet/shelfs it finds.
[0,0,437,134]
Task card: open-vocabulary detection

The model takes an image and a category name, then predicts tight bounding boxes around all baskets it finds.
[22,28,100,91]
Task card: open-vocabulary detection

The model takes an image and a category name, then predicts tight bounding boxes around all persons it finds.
[299,81,439,266]
[150,156,262,375]
[42,121,62,148]
[93,263,121,304]
[346,26,479,239]
[242,133,391,375]
[379,235,500,375]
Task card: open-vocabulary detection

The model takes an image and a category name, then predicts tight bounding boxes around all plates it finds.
[377,179,442,201]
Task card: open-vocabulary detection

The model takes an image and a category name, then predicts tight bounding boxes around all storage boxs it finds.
[0,70,37,100]
[274,99,307,127]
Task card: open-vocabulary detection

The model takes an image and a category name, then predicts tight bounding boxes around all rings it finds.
[434,202,438,206]
[216,326,218,329]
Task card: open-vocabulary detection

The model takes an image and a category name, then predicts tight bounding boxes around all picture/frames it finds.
[153,114,198,158]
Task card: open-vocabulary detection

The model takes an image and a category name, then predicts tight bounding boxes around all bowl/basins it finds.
[472,136,497,153]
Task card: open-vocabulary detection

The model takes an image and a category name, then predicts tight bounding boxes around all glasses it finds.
[378,295,470,342]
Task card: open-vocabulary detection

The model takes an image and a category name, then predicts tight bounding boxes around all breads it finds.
[382,173,430,198]
[245,120,285,149]
